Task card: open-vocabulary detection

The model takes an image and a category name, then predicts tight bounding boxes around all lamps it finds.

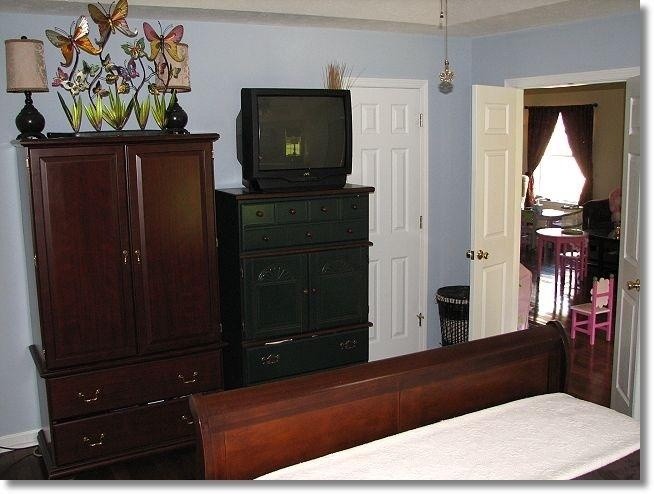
[146,33,194,137]
[3,32,54,140]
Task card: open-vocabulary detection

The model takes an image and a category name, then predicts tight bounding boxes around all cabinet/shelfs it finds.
[213,178,378,389]
[7,131,232,478]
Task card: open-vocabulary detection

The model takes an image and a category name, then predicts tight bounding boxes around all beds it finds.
[184,317,641,480]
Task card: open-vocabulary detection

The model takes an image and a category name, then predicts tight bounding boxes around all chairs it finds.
[581,196,621,266]
[559,239,587,282]
[519,207,535,250]
[569,273,616,347]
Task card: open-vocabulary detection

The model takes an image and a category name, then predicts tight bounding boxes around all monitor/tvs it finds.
[236,87,353,195]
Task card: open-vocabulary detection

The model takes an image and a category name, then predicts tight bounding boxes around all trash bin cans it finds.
[436,285,470,346]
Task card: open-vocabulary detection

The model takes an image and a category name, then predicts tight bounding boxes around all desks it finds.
[524,205,583,256]
[585,225,619,268]
[534,225,587,288]
